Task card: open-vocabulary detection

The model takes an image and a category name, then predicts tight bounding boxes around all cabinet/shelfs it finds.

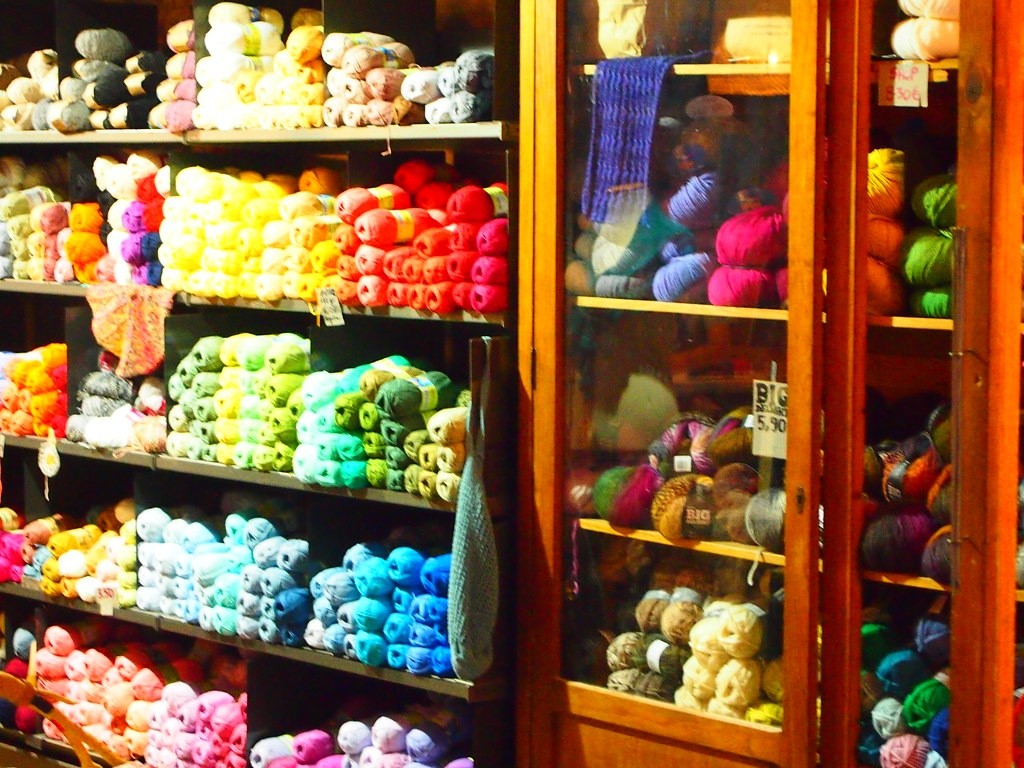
[0,2,535,768]
[534,0,1024,768]
